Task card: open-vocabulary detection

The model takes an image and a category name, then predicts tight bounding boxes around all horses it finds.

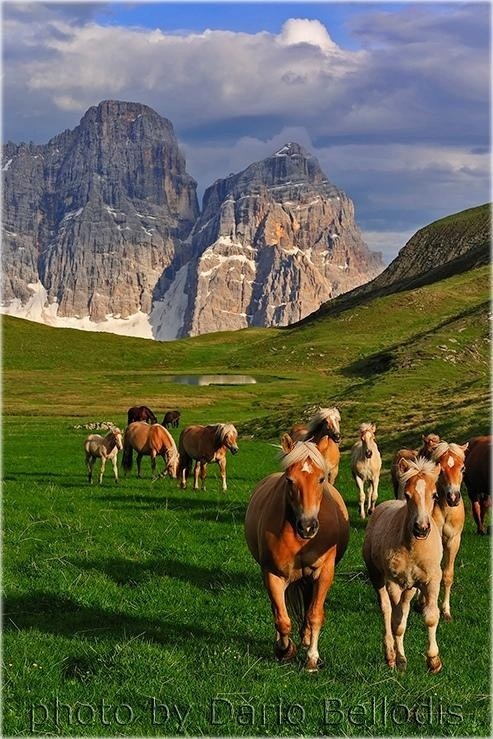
[83,423,124,486]
[127,405,157,426]
[176,422,238,492]
[240,402,492,673]
[121,420,180,484]
[160,411,181,430]
